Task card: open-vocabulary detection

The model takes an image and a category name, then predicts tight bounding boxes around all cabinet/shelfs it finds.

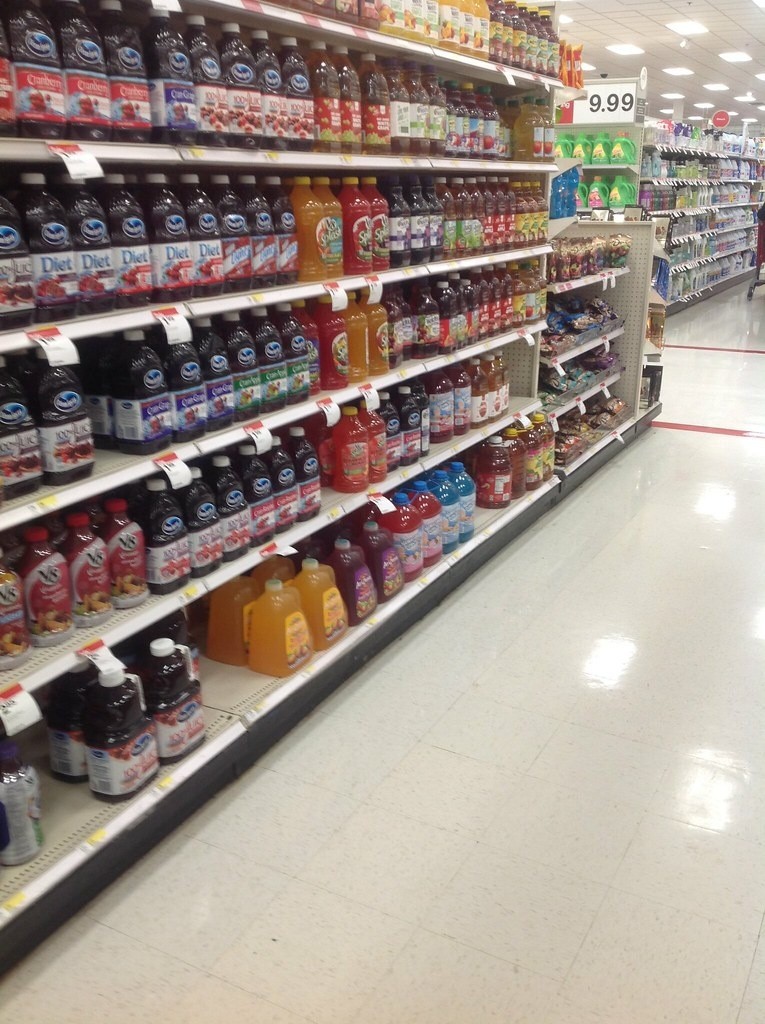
[0,0,765,1024]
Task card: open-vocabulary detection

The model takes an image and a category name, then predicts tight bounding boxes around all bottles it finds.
[575,175,638,208]
[554,131,636,164]
[0,171,554,866]
[640,120,765,302]
[0,0,562,164]
[549,166,578,219]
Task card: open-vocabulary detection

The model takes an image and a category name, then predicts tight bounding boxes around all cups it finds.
[548,234,632,284]
